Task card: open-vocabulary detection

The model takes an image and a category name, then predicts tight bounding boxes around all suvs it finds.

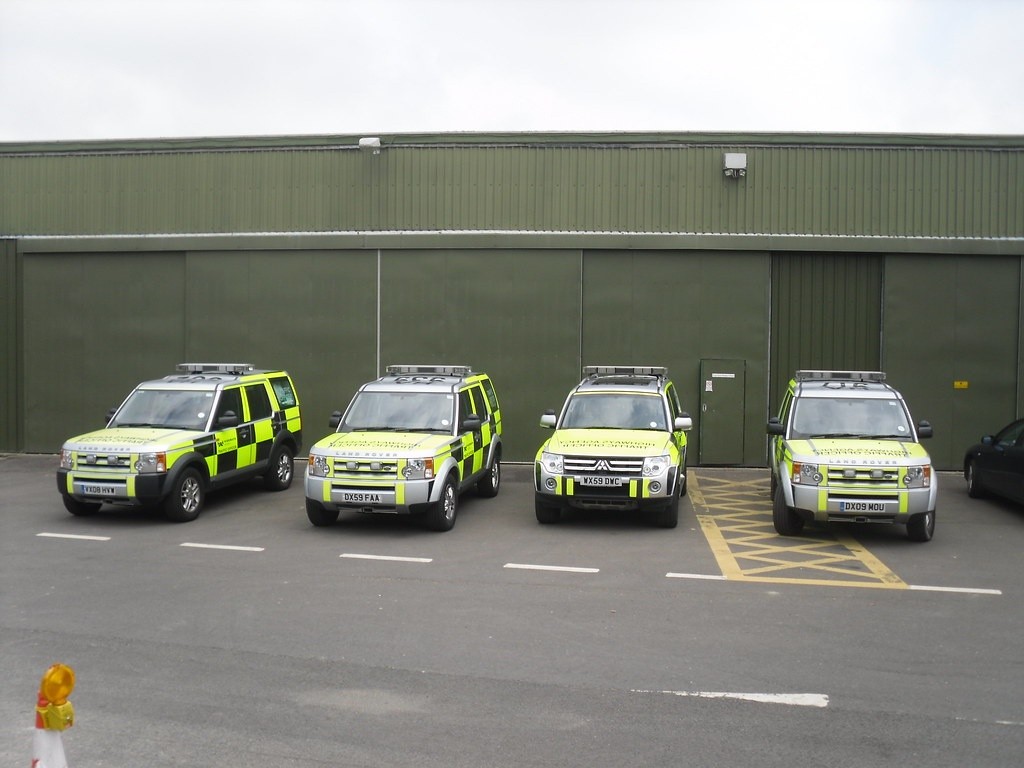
[56,363,302,521]
[304,366,503,532]
[534,366,692,529]
[766,368,937,542]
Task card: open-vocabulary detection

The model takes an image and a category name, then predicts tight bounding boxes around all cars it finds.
[962,417,1024,517]
